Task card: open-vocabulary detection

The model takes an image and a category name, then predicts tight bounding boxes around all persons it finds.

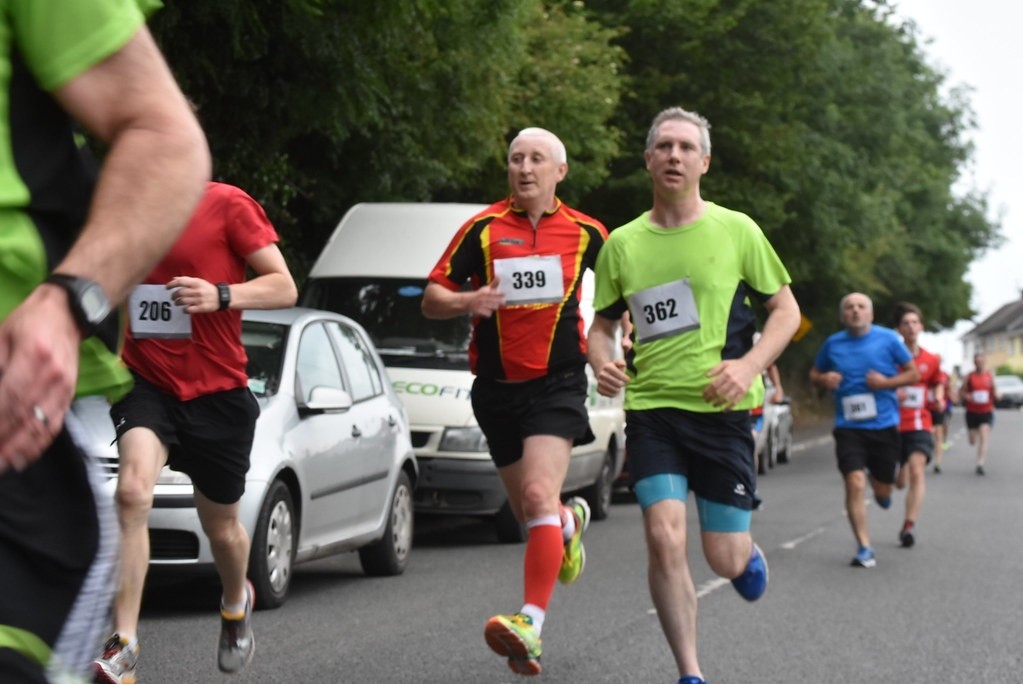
[888,302,1003,547]
[0,0,213,684]
[420,128,632,677]
[587,108,802,683]
[747,329,786,481]
[809,293,921,567]
[89,100,297,683]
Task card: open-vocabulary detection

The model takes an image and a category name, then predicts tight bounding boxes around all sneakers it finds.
[484,611,543,675]
[874,493,891,508]
[85,634,140,684]
[218,579,255,674]
[557,496,590,585]
[850,547,876,567]
[677,676,706,684]
[732,542,769,602]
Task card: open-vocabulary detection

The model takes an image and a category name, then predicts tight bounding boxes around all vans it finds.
[294,201,628,537]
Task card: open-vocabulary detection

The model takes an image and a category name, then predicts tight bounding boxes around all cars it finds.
[749,363,793,474]
[93,306,419,610]
[993,375,1023,406]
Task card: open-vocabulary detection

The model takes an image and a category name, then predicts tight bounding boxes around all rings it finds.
[32,404,49,429]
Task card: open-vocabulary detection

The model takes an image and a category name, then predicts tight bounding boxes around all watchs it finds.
[215,282,232,314]
[43,273,113,342]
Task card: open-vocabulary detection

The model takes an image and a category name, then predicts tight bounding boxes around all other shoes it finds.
[934,466,941,472]
[978,466,984,475]
[942,442,948,450]
[901,530,914,547]
